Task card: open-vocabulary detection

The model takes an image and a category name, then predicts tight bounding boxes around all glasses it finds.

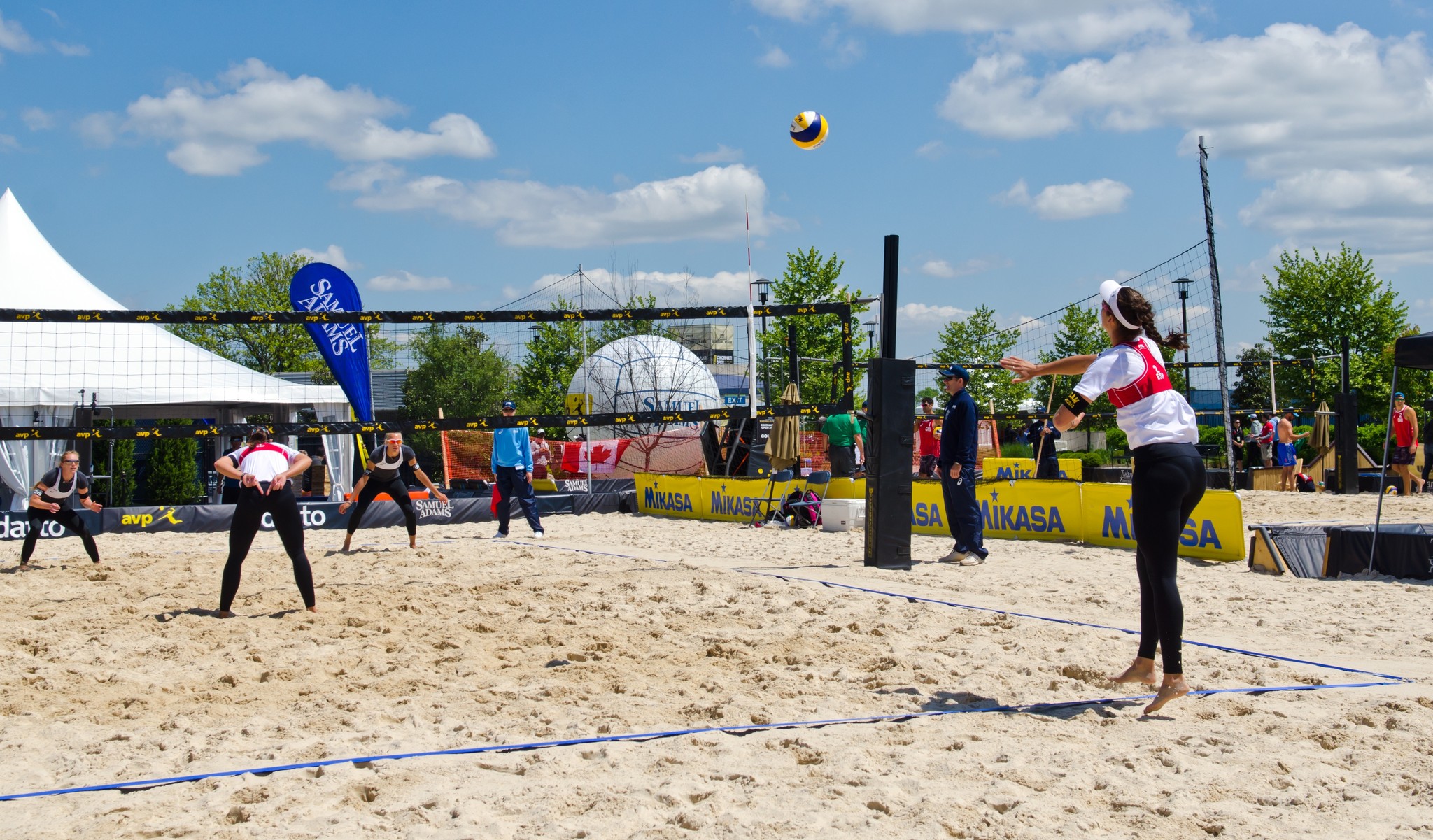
[232,439,242,441]
[1235,422,1241,424]
[64,460,80,466]
[1102,299,1113,314]
[921,404,930,407]
[945,376,956,381]
[863,407,867,411]
[387,439,403,445]
[538,434,545,436]
[1396,399,1405,401]
[502,409,515,412]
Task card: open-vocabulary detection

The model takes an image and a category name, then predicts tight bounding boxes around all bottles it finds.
[1318,481,1324,487]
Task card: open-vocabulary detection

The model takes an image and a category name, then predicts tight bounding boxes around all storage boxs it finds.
[820,498,866,532]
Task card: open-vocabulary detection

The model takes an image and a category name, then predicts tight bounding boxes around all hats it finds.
[1288,406,1299,417]
[938,365,970,381]
[1036,407,1047,413]
[1100,280,1143,330]
[1248,414,1257,418]
[573,434,584,440]
[537,429,544,434]
[251,427,271,438]
[1395,392,1405,398]
[862,401,868,408]
[501,401,516,409]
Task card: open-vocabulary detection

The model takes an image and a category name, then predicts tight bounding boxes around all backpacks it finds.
[1294,473,1316,492]
[800,489,823,527]
[783,490,805,523]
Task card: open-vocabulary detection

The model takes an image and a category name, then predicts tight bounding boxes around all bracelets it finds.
[823,450,827,453]
[346,501,352,505]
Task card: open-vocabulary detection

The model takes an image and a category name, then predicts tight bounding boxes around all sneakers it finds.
[938,549,969,562]
[959,551,985,565]
[492,532,507,538]
[534,530,543,539]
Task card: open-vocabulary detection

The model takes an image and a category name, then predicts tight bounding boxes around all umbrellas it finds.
[1307,399,1330,484]
[763,380,799,526]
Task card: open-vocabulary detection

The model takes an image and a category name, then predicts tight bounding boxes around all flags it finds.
[560,439,632,474]
[491,483,502,518]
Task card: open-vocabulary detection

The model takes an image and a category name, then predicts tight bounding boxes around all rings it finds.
[1012,366,1015,372]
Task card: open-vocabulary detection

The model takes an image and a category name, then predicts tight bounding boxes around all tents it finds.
[1367,331,1433,576]
[917,391,1047,434]
[0,188,352,510]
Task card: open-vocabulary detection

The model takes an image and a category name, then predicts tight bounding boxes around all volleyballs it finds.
[563,335,721,479]
[783,515,800,529]
[1386,485,1397,495]
[790,110,830,150]
[933,426,942,440]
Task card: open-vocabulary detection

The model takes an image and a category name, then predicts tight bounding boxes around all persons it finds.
[491,400,587,539]
[20,450,103,568]
[938,365,989,566]
[214,426,325,618]
[1232,393,1433,497]
[1003,408,1062,481]
[822,398,943,480]
[336,432,448,552]
[1000,279,1207,715]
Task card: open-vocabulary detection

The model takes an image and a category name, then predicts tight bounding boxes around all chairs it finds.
[789,471,831,529]
[748,469,794,527]
[1272,457,1303,492]
